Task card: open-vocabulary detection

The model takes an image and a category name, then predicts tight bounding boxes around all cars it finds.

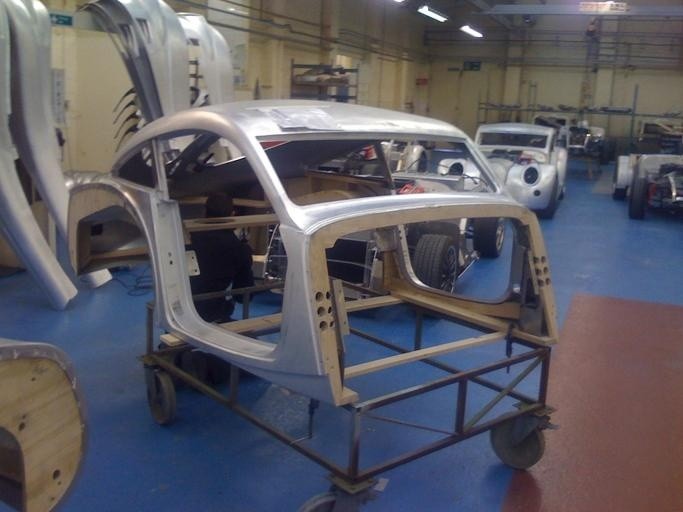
[231,139,507,324]
[611,152,683,220]
[556,119,605,160]
[464,122,569,219]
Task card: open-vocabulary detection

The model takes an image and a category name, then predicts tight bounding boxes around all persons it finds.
[183,192,260,323]
